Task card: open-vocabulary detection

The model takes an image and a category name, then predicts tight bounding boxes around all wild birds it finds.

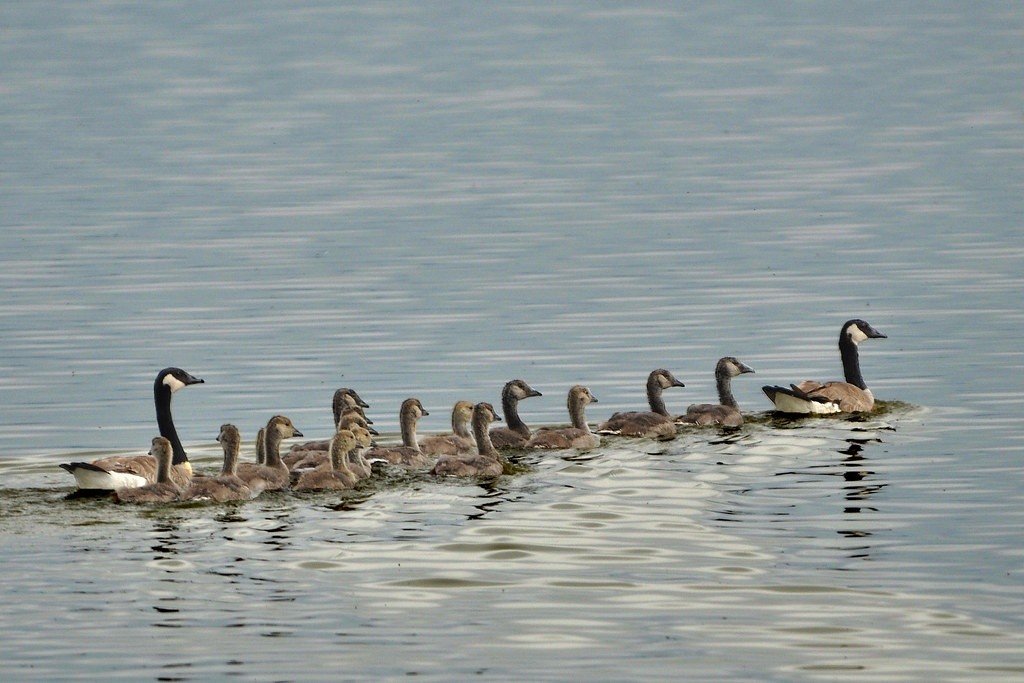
[523,383,602,451]
[762,319,889,415]
[594,368,685,436]
[111,436,184,504]
[187,378,544,505]
[672,356,758,427]
[58,366,205,498]
